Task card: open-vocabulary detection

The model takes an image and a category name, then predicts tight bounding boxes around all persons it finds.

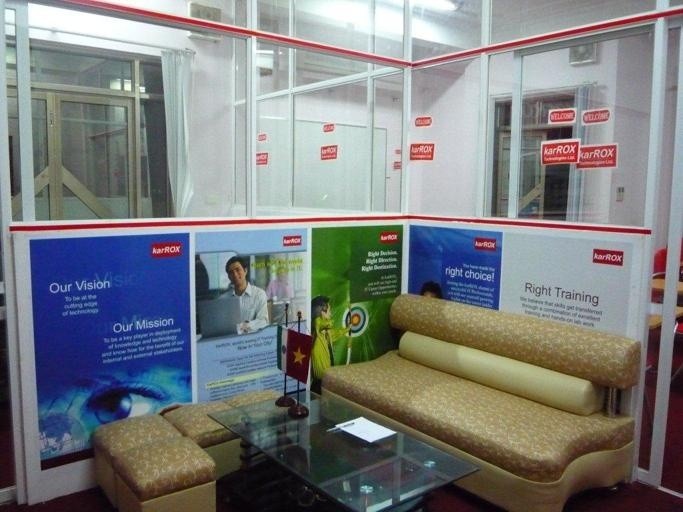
[420,281,443,301]
[194,252,210,337]
[263,265,295,301]
[310,293,351,397]
[215,255,271,335]
[40,364,193,453]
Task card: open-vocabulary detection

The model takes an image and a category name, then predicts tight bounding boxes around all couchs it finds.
[320,293,641,512]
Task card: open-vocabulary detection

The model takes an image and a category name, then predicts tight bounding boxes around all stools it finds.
[162,399,249,481]
[111,436,216,512]
[92,413,183,509]
[221,389,285,409]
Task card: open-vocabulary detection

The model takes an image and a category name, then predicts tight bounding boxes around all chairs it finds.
[645,237,683,440]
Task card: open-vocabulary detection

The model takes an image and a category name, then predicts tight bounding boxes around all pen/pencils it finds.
[326,421,355,433]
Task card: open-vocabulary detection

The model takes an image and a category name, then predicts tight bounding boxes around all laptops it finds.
[198,296,243,338]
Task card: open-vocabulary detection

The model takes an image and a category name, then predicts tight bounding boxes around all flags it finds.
[286,328,312,384]
[276,324,288,375]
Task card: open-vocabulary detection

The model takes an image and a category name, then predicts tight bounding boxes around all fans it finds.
[569,44,596,66]
